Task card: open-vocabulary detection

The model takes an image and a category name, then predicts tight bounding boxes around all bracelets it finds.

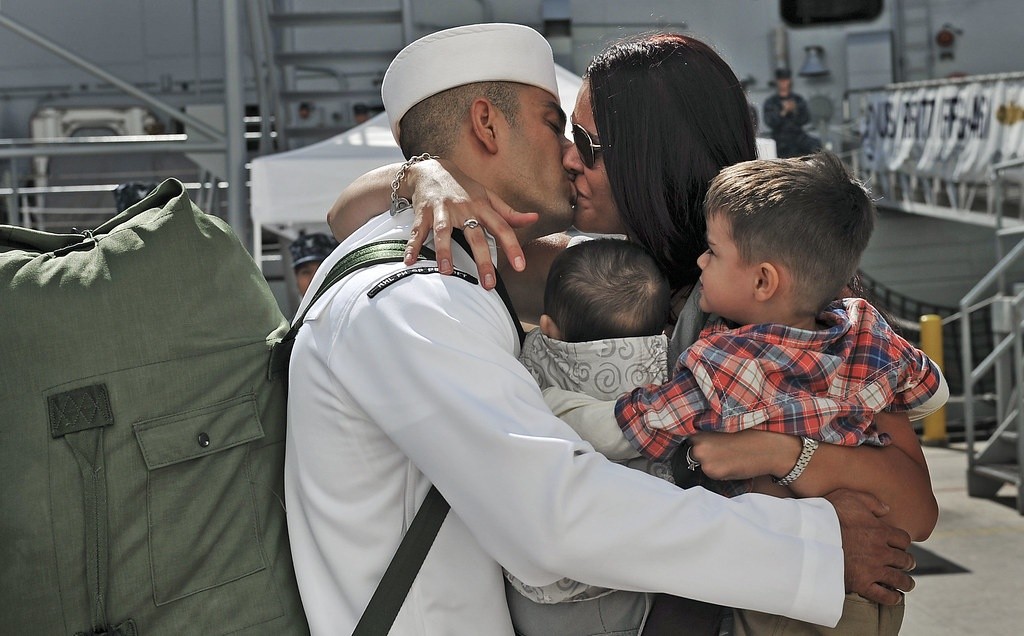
[388,151,440,215]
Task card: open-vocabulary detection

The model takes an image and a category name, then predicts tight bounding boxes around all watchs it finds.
[774,433,820,488]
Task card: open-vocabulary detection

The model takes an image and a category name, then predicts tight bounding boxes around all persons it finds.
[325,32,944,636]
[537,156,952,636]
[278,19,919,634]
[522,237,682,634]
[762,67,817,156]
[290,230,341,296]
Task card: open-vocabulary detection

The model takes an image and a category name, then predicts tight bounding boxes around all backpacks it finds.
[0,175,523,635]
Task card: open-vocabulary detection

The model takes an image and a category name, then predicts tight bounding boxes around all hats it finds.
[775,68,792,81]
[289,233,338,268]
[380,24,561,152]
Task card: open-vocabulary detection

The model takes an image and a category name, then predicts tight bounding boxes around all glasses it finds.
[571,123,613,169]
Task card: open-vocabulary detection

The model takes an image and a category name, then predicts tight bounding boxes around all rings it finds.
[460,217,482,232]
[685,444,701,472]
